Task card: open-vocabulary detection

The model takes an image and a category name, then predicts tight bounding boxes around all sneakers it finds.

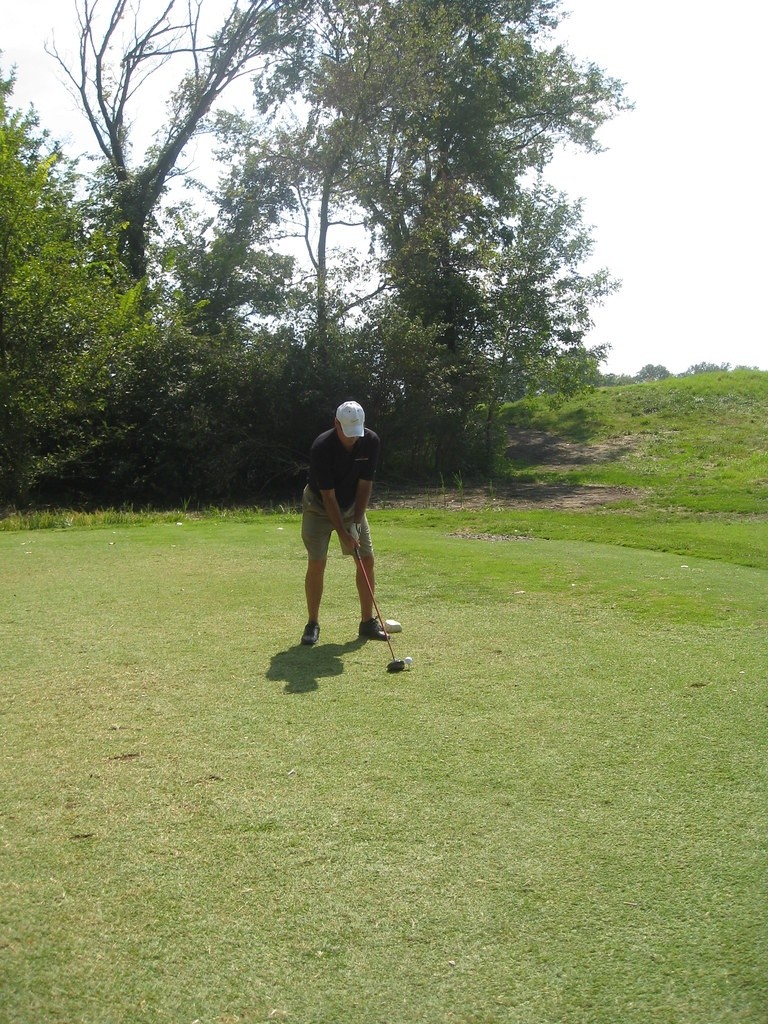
[301,623,319,645]
[359,618,390,641]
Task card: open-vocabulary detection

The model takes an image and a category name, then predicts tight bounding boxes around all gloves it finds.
[348,522,361,543]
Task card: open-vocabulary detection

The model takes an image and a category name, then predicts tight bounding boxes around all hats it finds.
[336,400,365,437]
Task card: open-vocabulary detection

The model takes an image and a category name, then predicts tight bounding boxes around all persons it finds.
[301,401,390,645]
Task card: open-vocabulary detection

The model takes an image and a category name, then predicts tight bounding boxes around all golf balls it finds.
[405,656,413,664]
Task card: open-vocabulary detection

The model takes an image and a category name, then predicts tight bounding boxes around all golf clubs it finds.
[356,545,404,673]
